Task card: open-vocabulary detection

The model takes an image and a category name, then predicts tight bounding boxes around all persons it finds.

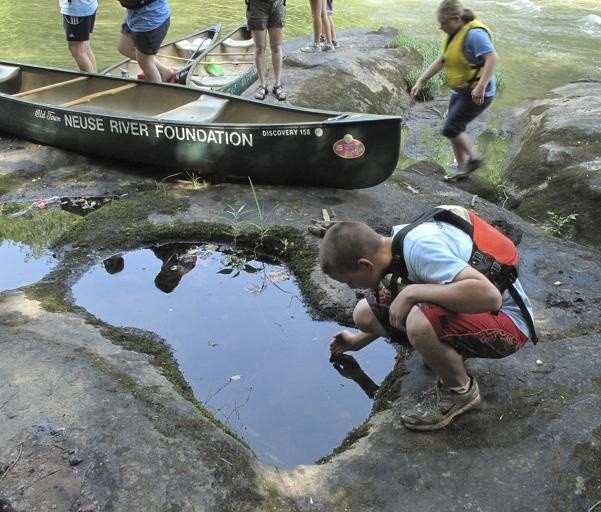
[115,0,175,84]
[243,0,290,101]
[57,0,98,74]
[104,255,125,275]
[317,202,537,433]
[300,1,336,54]
[409,0,502,183]
[319,1,340,47]
[329,352,380,399]
[153,243,198,293]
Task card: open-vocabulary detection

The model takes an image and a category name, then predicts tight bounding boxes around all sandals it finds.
[255,84,268,100]
[273,84,287,101]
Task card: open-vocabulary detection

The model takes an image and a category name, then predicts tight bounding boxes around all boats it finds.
[185,22,269,97]
[97,20,224,83]
[37,66,402,191]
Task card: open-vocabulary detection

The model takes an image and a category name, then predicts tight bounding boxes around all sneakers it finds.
[444,154,484,182]
[301,35,340,52]
[400,373,481,431]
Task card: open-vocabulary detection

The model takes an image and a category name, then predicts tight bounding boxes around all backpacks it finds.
[391,205,519,295]
